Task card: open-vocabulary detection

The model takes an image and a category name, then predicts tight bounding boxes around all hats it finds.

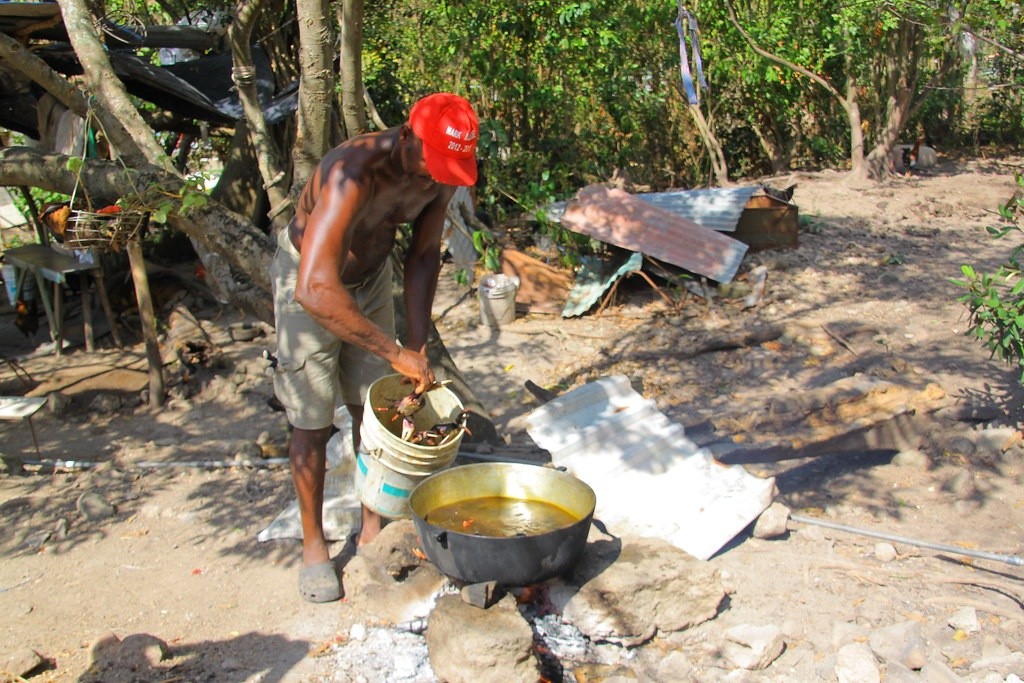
[409,92,479,186]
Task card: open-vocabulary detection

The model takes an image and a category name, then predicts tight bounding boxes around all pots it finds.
[407,462,596,587]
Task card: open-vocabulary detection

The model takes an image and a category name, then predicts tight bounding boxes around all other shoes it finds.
[299,561,344,603]
[348,531,396,582]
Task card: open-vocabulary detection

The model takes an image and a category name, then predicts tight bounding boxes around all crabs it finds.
[375,380,475,446]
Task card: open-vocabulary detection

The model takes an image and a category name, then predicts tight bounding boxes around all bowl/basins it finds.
[229,321,260,341]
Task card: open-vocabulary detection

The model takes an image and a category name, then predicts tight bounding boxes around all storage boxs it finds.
[727,194,800,252]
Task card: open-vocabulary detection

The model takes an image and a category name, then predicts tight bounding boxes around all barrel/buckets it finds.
[478,273,517,326]
[353,373,467,522]
[2,265,35,305]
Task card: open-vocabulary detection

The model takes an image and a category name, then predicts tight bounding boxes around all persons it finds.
[268,91,480,605]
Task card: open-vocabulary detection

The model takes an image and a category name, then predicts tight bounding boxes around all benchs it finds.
[0,395,49,459]
[2,242,121,358]
